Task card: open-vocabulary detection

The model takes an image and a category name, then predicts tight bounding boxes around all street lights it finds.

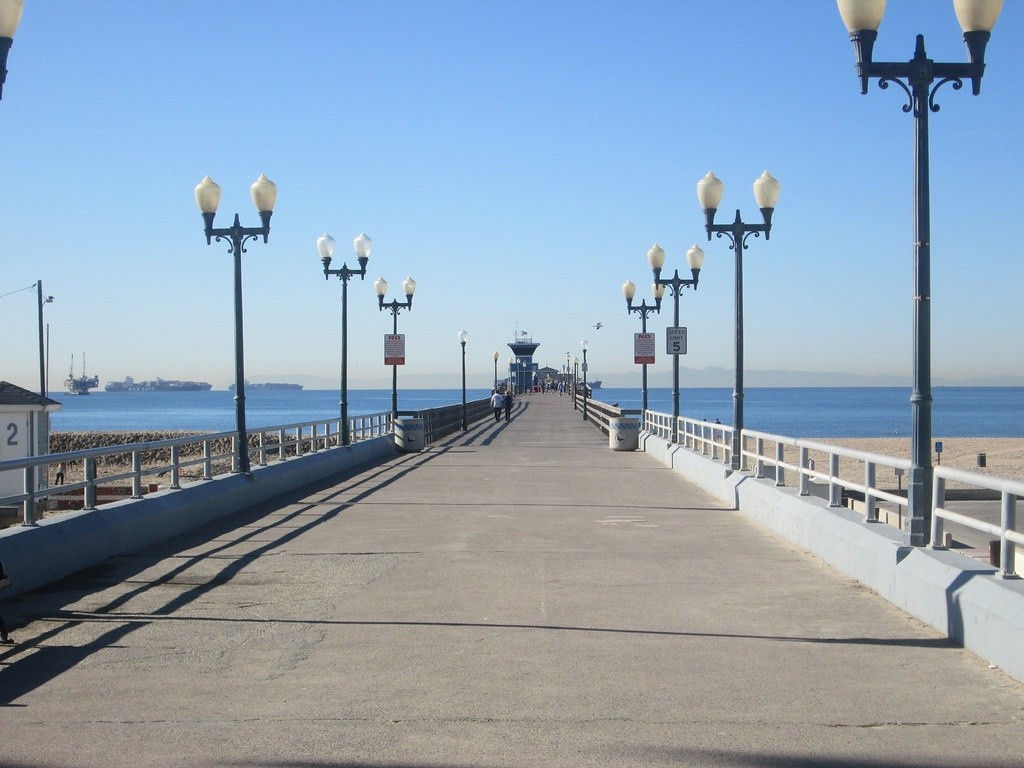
[573,357,580,410]
[317,231,373,445]
[493,351,500,394]
[509,358,513,398]
[624,278,667,436]
[376,276,414,424]
[196,169,277,478]
[836,0,1002,550]
[647,242,707,444]
[458,328,469,434]
[580,339,590,421]
[696,169,782,469]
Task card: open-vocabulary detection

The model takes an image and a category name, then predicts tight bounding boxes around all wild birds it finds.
[593,322,603,330]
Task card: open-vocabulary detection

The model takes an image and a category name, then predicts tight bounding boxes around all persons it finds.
[502,391,513,422]
[538,379,567,398]
[703,418,723,441]
[491,391,504,420]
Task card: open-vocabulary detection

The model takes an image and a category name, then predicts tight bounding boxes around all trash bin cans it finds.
[609,417,639,451]
[395,419,424,454]
